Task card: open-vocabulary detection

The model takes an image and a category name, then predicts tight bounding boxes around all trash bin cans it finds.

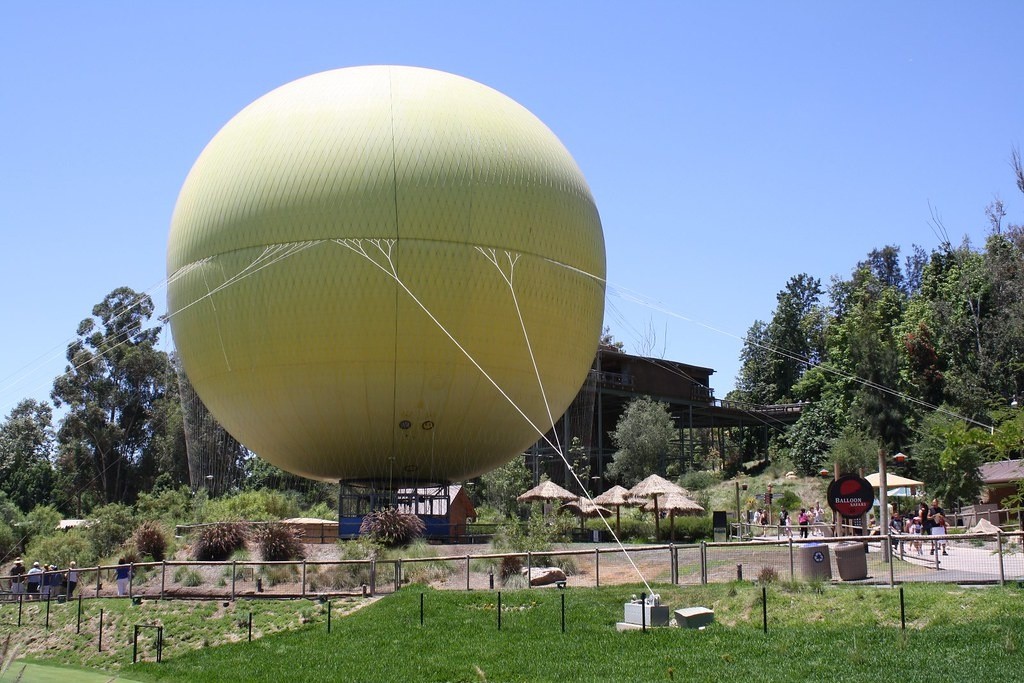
[798,541,832,581]
[594,530,602,542]
[834,542,868,580]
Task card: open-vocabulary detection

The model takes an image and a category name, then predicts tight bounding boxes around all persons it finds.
[887,499,948,555]
[807,506,817,536]
[110,558,135,596]
[10,558,81,600]
[798,508,810,538]
[754,509,768,537]
[813,502,825,536]
[777,505,793,537]
[868,514,875,528]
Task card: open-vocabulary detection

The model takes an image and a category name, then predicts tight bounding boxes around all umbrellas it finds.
[516,479,580,516]
[556,496,613,538]
[622,474,705,542]
[587,485,648,540]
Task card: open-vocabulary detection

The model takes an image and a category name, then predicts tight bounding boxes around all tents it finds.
[864,472,924,494]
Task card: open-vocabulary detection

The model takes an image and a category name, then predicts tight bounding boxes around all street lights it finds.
[818,463,844,545]
[192,473,214,514]
[734,481,749,542]
[766,483,774,524]
[877,447,908,563]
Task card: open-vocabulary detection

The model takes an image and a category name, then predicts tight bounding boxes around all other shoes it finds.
[943,551,948,555]
[931,549,935,555]
[937,548,940,550]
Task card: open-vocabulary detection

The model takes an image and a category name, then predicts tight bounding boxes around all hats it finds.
[53,566,58,570]
[14,559,23,563]
[34,562,39,566]
